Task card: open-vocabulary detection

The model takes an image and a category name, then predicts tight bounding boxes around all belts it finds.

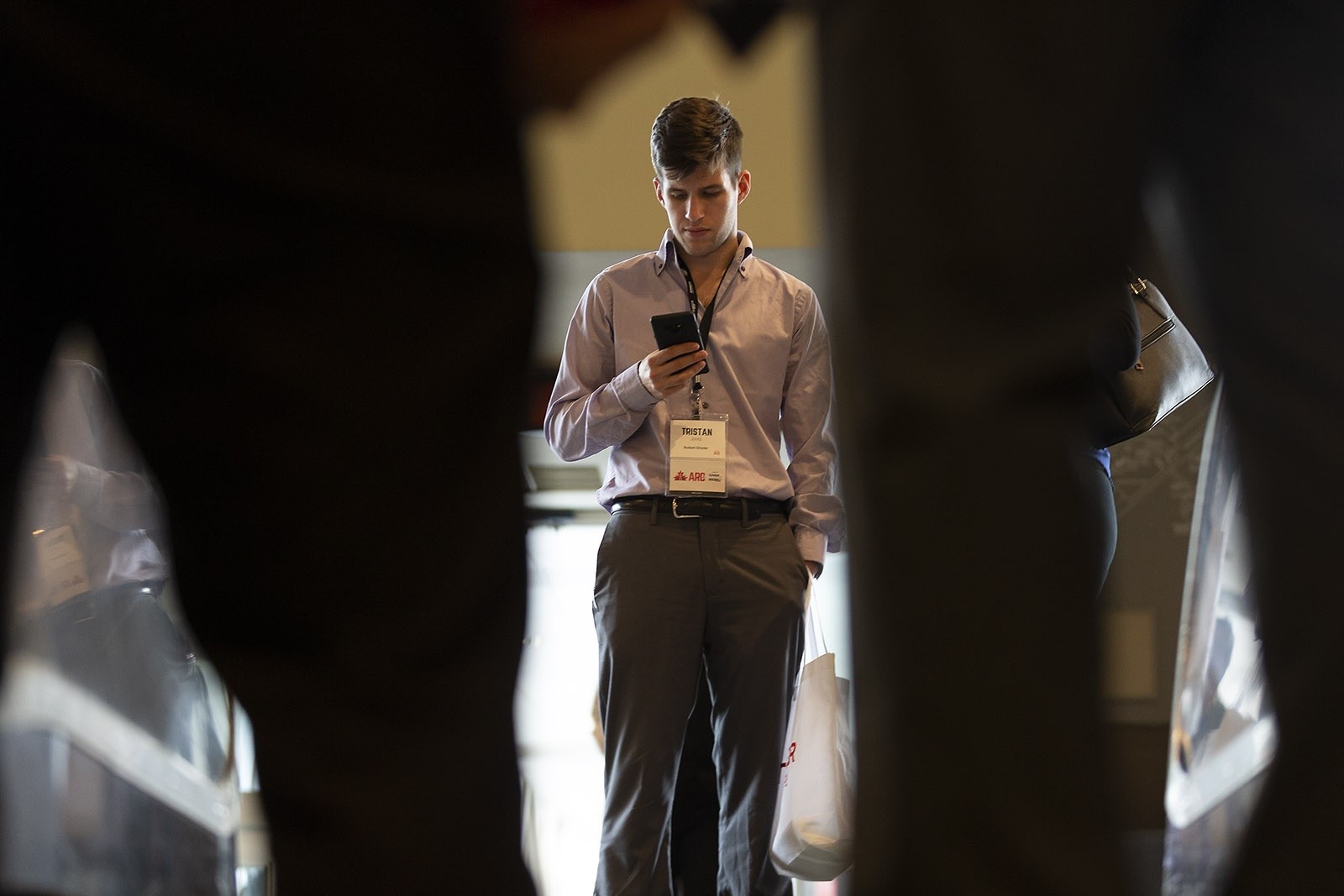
[13,580,154,652]
[610,492,788,520]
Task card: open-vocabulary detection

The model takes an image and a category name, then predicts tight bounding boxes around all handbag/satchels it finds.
[769,574,856,881]
[1075,263,1215,451]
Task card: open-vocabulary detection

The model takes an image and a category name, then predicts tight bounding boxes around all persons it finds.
[0,0,1344,896]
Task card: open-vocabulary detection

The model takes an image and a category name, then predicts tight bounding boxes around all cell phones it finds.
[649,310,709,375]
